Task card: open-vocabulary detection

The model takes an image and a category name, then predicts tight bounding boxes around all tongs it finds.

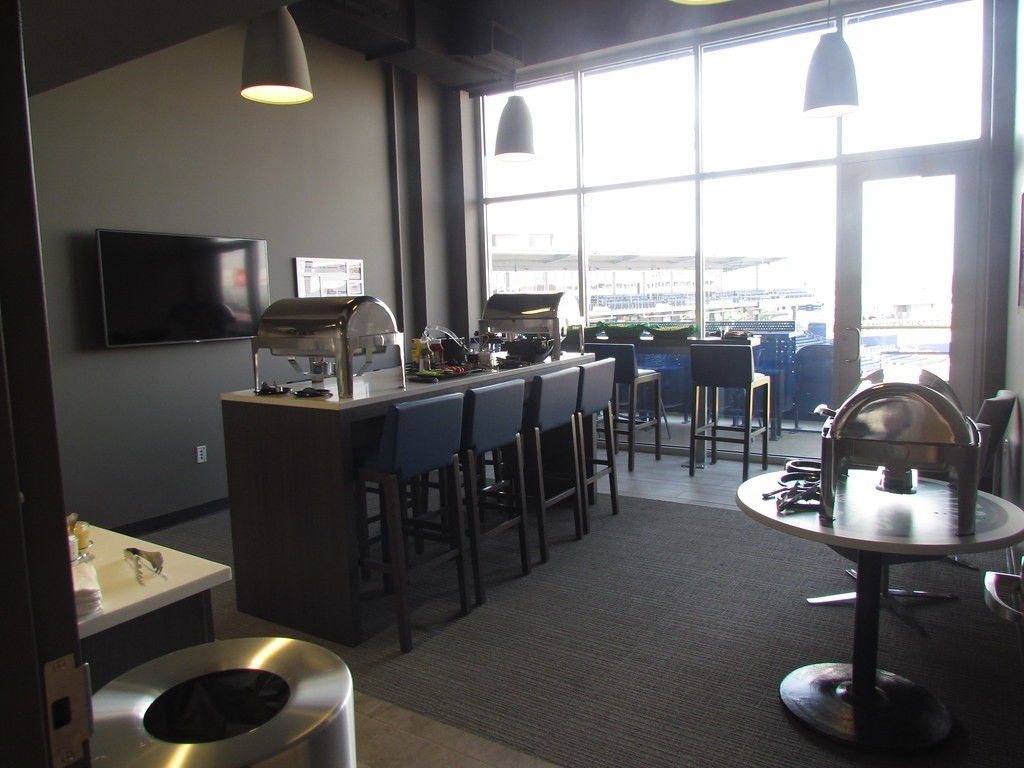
[776,480,820,511]
[123,547,164,586]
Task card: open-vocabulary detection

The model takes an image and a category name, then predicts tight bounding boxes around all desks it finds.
[223,348,595,648]
[736,470,1024,754]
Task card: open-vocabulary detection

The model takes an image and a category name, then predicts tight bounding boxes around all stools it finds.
[356,341,772,651]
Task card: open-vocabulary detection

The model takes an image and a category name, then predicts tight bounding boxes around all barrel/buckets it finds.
[436,335,466,364]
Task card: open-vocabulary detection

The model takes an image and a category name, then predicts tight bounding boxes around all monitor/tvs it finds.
[96,228,271,348]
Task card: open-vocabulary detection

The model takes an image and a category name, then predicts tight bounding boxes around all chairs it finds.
[949,389,1016,482]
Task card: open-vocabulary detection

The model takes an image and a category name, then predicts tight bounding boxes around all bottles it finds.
[467,348,490,365]
[418,339,443,372]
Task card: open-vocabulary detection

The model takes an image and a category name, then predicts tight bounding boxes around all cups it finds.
[68,520,90,554]
[719,325,729,338]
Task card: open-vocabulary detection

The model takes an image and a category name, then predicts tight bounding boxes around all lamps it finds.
[240,0,313,105]
[493,40,537,163]
[804,0,857,116]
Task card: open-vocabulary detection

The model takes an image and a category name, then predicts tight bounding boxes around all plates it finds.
[777,459,821,511]
[724,335,754,339]
[293,389,330,398]
[253,387,292,395]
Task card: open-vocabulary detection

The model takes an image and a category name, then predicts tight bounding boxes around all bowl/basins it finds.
[504,337,555,364]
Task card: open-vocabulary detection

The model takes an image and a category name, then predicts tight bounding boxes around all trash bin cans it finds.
[88,637,358,768]
